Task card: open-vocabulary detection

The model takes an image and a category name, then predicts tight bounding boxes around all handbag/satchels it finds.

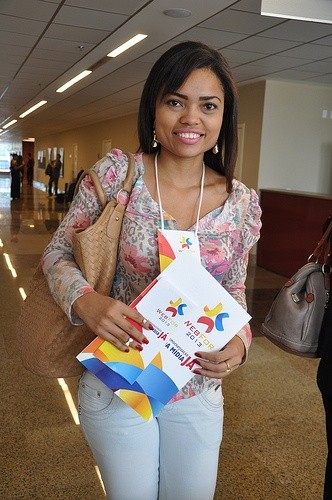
[18,152,135,377]
[260,219,332,356]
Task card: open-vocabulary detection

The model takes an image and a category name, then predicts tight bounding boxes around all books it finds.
[75,248,252,424]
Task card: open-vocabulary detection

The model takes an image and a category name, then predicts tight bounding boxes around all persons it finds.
[10,153,24,200]
[45,154,62,198]
[41,41,262,500]
[26,152,34,185]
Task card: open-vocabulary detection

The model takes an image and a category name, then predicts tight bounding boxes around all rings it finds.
[125,338,133,347]
[139,318,147,327]
[225,361,232,375]
